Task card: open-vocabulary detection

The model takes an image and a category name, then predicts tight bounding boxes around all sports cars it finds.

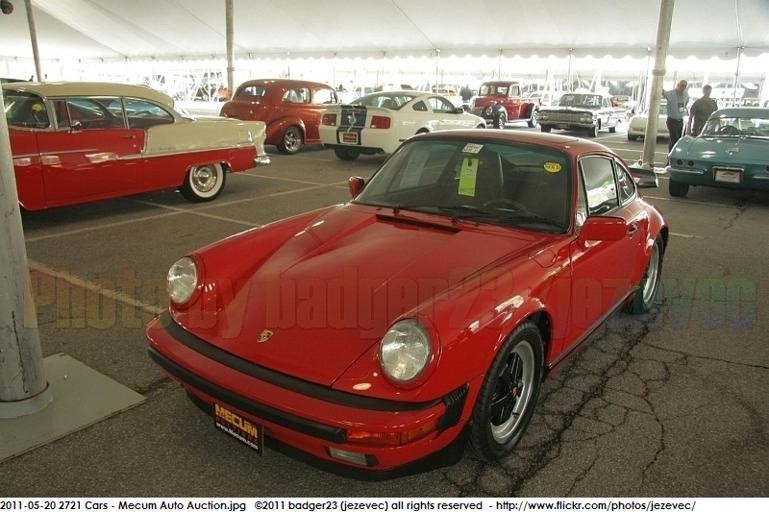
[317,90,489,162]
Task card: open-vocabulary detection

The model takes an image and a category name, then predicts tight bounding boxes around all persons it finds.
[687,85,718,138]
[661,79,690,166]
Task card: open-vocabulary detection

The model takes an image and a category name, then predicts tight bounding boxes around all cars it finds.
[666,101,768,195]
[218,76,339,156]
[627,101,695,143]
[147,130,672,489]
[535,89,624,136]
[461,79,541,131]
[2,78,271,214]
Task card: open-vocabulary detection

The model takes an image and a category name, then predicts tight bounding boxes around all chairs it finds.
[450,151,504,212]
